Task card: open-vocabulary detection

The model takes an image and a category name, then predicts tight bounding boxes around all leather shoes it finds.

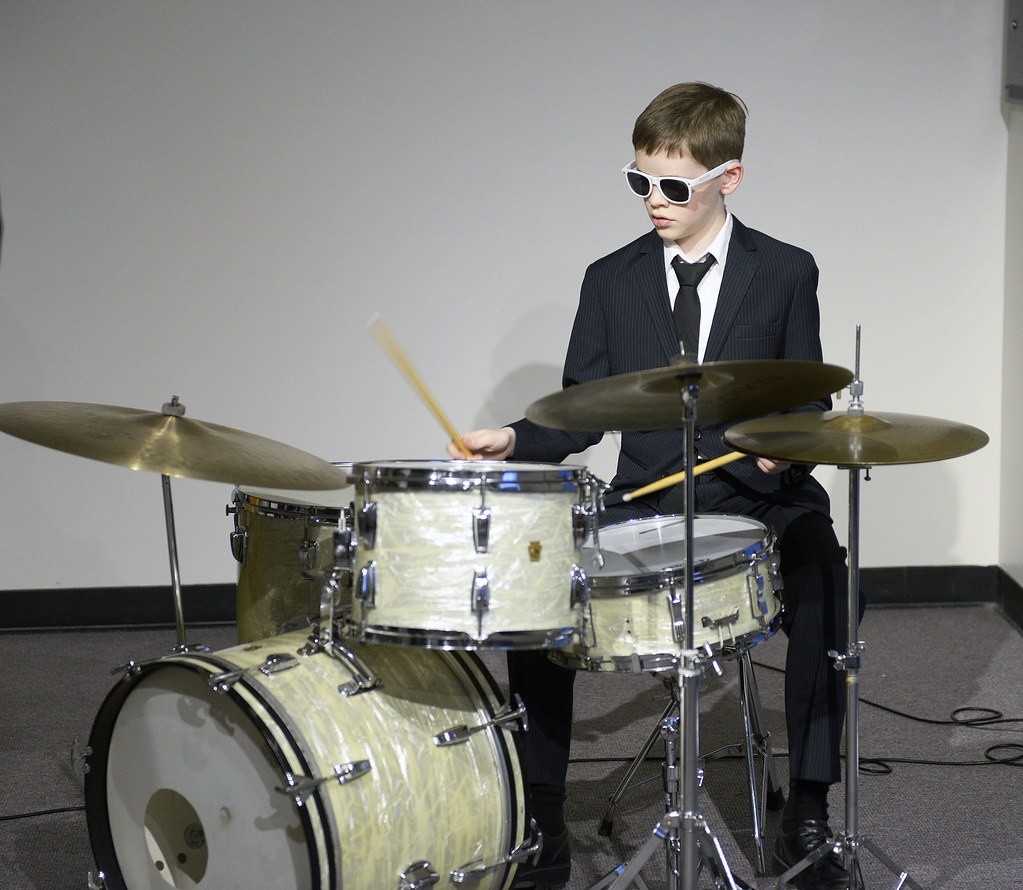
[771,818,852,890]
[511,828,571,884]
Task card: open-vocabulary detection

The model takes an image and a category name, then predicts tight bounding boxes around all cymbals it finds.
[719,400,994,469]
[1,390,351,495]
[523,352,855,435]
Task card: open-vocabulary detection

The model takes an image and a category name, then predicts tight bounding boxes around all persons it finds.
[447,81,867,890]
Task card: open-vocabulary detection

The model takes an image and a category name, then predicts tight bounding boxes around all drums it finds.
[345,454,590,656]
[230,460,362,646]
[79,620,527,890]
[546,513,788,676]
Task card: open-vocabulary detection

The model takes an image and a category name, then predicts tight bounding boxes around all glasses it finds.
[622,159,741,205]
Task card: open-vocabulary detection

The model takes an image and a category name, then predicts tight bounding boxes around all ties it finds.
[671,253,716,363]
